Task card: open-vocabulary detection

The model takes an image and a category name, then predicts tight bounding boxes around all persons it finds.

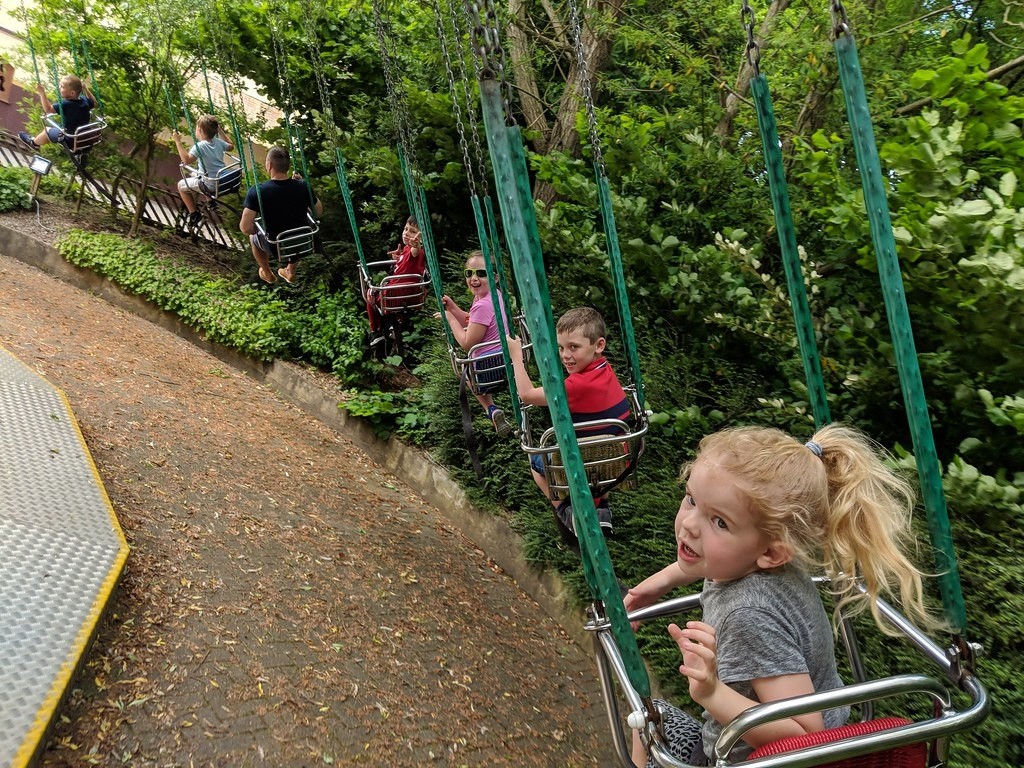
[364,213,427,349]
[620,426,959,767]
[502,306,637,541]
[432,249,511,439]
[17,75,98,154]
[237,145,324,285]
[171,115,234,223]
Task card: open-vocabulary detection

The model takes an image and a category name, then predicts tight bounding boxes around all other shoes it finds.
[207,200,220,210]
[76,153,89,173]
[18,131,40,153]
[488,404,514,440]
[370,331,385,346]
[188,211,203,227]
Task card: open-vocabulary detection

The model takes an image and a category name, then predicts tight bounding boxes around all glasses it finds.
[464,268,498,279]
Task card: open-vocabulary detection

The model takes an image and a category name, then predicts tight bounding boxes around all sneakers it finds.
[564,505,579,537]
[278,268,295,284]
[258,267,277,283]
[597,507,613,536]
